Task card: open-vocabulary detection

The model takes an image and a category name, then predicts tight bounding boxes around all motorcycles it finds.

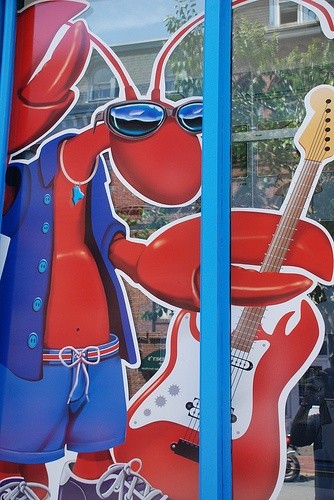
[284,434,302,482]
